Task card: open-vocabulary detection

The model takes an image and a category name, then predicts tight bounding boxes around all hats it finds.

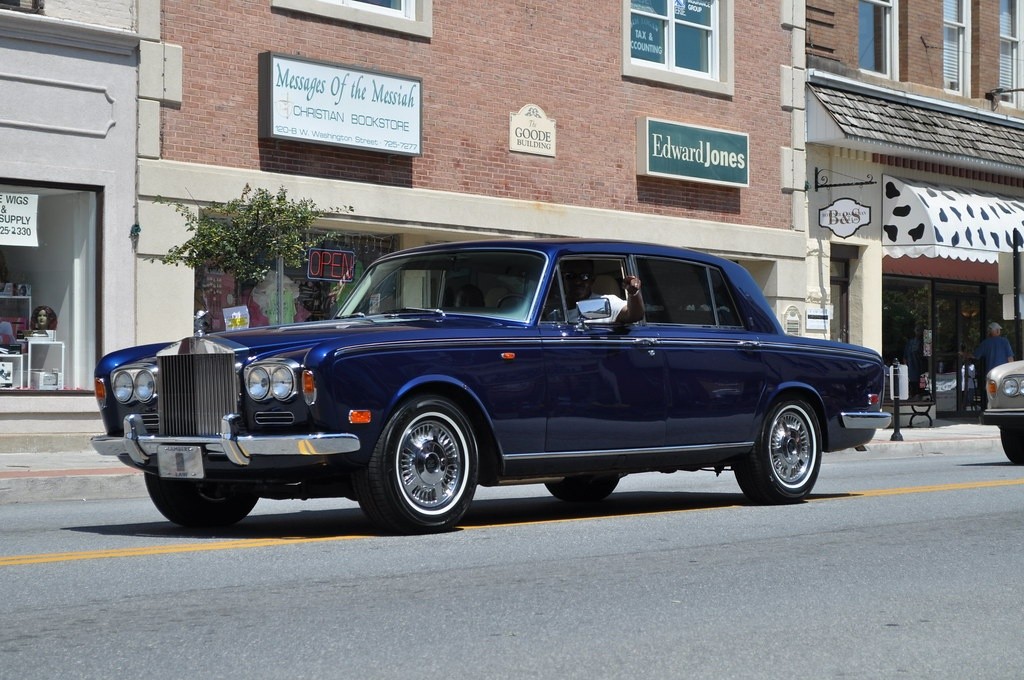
[987,322,1003,331]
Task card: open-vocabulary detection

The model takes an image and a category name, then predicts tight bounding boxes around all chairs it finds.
[483,286,511,310]
[596,275,622,297]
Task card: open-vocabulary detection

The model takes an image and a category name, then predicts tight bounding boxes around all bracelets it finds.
[628,290,640,296]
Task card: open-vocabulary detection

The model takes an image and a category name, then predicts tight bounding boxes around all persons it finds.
[542,260,645,325]
[903,320,928,398]
[973,322,1015,424]
[30,305,57,330]
[453,285,486,310]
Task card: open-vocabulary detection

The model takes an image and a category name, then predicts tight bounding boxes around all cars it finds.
[981,358,1024,464]
[88,239,892,533]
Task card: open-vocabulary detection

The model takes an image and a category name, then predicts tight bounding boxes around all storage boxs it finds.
[31,370,64,391]
[24,330,56,341]
[0,283,31,296]
[0,361,14,384]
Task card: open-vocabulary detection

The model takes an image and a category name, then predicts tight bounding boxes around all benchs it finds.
[883,370,936,428]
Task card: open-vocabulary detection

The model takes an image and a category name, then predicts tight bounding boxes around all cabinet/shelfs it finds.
[0,295,65,391]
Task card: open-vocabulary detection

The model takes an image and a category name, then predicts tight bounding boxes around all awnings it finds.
[882,174,1024,264]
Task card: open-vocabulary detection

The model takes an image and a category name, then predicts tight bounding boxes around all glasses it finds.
[563,271,593,281]
